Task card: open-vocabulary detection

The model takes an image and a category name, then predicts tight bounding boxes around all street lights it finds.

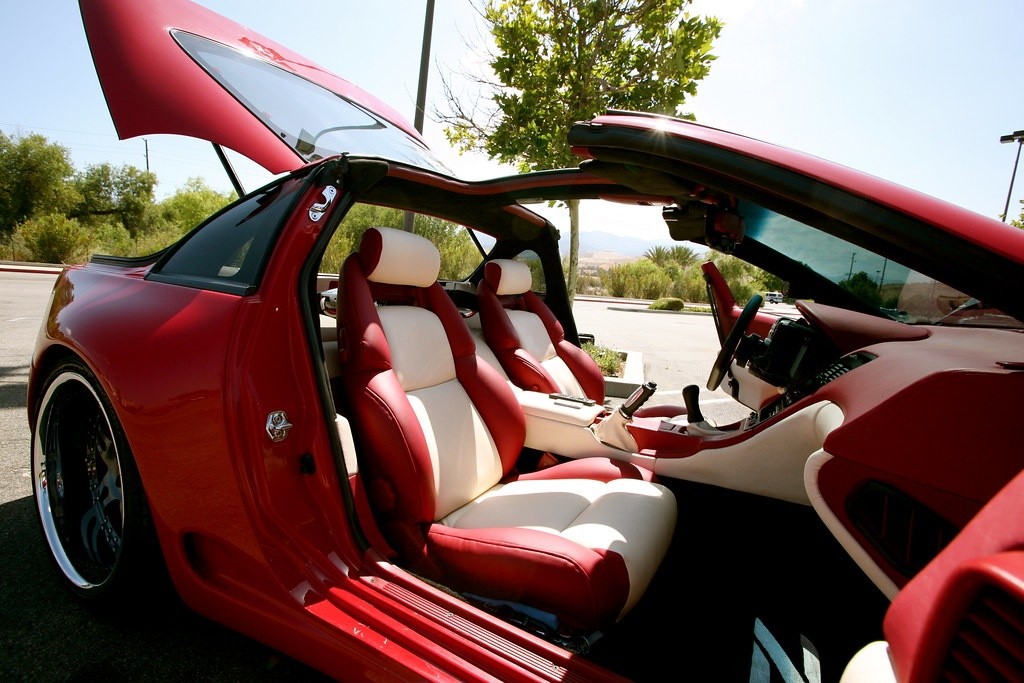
[999,130,1024,221]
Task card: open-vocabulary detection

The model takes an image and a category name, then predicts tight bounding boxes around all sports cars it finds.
[26,1,1023,683]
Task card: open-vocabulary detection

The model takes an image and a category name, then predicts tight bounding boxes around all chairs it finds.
[336,227,677,638]
[478,258,703,450]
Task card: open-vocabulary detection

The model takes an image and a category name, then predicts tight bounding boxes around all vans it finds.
[765,292,783,304]
